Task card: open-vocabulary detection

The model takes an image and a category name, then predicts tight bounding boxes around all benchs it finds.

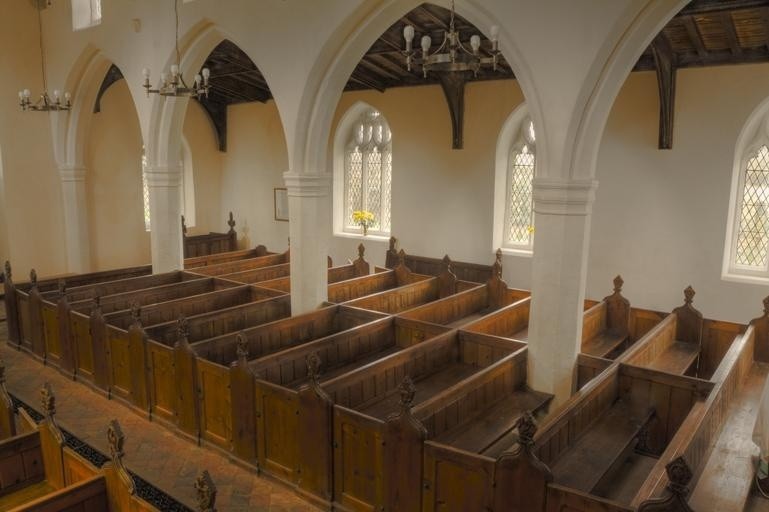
[4,210,769,509]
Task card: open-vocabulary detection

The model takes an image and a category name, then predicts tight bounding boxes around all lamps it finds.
[18,2,73,113]
[139,2,211,105]
[401,1,503,78]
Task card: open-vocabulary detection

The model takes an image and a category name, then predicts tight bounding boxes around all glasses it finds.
[139,2,211,105]
[18,2,73,113]
[401,1,503,78]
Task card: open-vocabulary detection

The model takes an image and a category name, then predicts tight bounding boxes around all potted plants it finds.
[273,187,290,221]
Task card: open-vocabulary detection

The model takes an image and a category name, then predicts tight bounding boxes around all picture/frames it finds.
[273,187,290,221]
